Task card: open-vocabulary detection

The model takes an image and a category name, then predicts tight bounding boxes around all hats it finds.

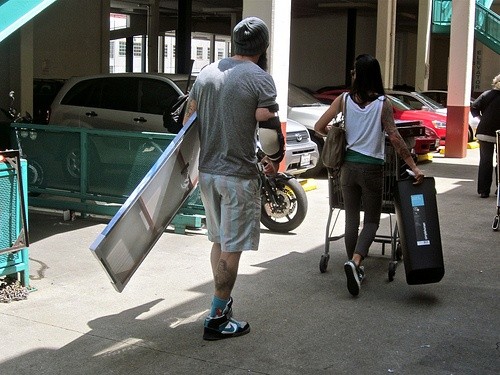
[232,17,271,56]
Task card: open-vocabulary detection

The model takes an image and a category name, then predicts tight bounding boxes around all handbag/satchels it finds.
[321,92,351,168]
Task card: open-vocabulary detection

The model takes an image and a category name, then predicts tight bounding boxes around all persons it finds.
[314,53,425,296]
[183,16,285,340]
[471,75,500,197]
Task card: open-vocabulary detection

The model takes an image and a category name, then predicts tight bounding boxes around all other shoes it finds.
[481,193,490,198]
[358,266,367,283]
[344,260,361,296]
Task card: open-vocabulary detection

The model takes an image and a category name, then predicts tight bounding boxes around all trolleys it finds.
[319,136,418,281]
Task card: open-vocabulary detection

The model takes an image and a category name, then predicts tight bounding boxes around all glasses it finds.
[351,69,357,75]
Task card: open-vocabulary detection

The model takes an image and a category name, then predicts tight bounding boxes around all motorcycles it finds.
[137,137,308,232]
[0,90,50,188]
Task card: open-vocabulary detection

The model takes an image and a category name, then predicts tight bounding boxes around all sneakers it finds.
[202,314,251,341]
[223,296,233,317]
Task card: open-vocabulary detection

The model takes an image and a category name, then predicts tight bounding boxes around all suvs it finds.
[45,72,320,186]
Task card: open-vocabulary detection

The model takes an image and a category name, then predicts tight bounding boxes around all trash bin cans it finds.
[0,156,32,291]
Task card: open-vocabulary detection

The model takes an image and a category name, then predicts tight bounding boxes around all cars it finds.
[316,90,446,150]
[289,82,345,145]
[401,91,481,141]
